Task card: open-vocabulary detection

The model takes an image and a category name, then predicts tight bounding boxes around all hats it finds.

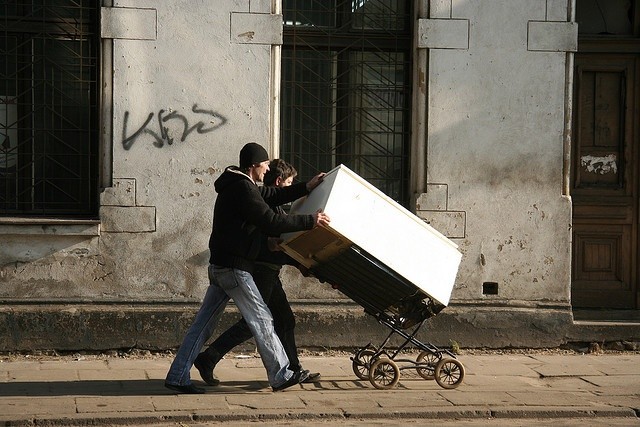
[240,143,269,165]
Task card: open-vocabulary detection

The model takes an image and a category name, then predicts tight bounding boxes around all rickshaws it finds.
[299,254,466,391]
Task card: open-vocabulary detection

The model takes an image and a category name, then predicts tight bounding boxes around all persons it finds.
[165,142,331,393]
[194,159,321,385]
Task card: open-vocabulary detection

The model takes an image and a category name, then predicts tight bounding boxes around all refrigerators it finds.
[280,163,463,322]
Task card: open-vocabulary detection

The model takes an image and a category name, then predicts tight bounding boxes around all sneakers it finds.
[272,369,308,393]
[163,380,206,393]
[292,366,321,382]
[193,355,220,386]
